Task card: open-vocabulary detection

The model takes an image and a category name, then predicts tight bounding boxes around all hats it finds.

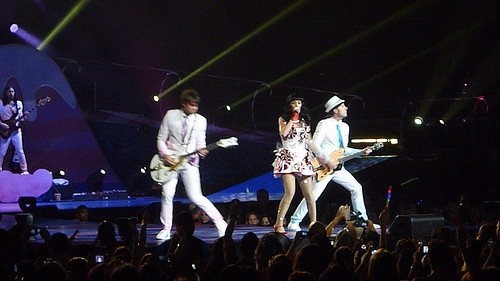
[325,96,345,113]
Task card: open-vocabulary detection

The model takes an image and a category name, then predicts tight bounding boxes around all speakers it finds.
[385,214,446,248]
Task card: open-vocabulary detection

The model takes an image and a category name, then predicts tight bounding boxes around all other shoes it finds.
[23,171,29,174]
[156,230,171,239]
[363,224,380,229]
[218,221,228,238]
[288,224,302,231]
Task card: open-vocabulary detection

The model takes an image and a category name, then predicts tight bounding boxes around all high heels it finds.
[274,225,288,235]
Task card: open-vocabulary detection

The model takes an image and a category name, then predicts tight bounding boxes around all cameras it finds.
[94,254,106,264]
[419,244,428,255]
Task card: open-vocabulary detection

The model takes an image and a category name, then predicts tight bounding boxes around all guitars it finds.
[312,141,384,183]
[149,136,239,183]
[0,96,52,140]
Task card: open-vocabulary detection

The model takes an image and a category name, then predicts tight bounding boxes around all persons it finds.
[0,189,500,281]
[272,93,325,235]
[287,96,381,232]
[0,86,29,173]
[156,90,228,240]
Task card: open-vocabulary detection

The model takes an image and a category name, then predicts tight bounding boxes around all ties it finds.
[337,125,346,153]
[183,118,187,141]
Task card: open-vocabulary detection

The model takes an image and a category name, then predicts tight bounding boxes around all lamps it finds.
[54,169,66,192]
[133,166,148,187]
[414,113,448,125]
[88,168,107,193]
